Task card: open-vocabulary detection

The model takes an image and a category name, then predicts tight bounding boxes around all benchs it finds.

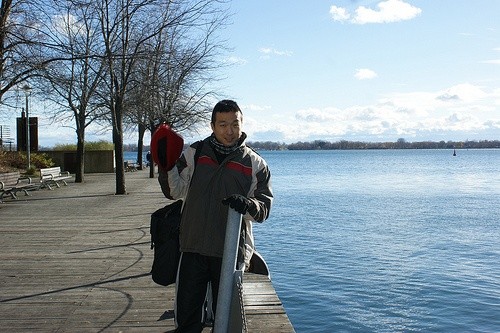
[0,172,34,200]
[127,160,140,172]
[37,167,72,190]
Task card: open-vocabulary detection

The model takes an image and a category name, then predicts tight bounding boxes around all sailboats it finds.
[453,148,456,155]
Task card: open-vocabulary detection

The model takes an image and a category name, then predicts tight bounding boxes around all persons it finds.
[146,151,151,167]
[156,99,274,333]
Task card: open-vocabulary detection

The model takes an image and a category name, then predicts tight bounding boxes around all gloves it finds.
[222,194,254,215]
[158,137,167,167]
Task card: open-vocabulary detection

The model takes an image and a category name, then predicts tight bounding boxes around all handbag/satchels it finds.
[150,200,183,286]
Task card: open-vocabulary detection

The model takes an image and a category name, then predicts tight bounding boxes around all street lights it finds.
[22,84,34,171]
